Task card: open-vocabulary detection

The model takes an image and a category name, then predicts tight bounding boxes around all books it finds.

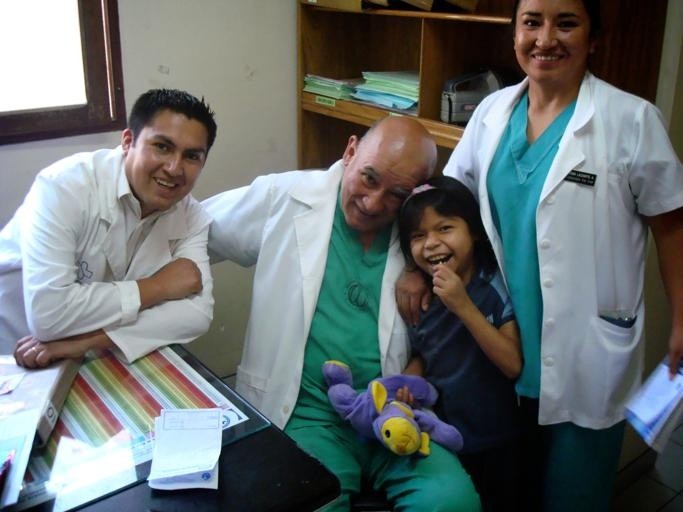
[624,355,683,454]
[0,351,81,451]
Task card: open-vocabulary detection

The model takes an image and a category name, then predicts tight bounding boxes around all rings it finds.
[31,346,37,355]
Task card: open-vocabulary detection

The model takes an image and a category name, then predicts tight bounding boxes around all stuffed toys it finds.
[319,356,463,458]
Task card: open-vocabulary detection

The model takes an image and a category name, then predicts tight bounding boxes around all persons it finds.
[199,114,485,512]
[390,175,525,512]
[0,87,216,370]
[394,0,683,509]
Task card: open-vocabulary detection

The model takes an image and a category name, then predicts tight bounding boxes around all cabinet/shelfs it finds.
[293,0,667,181]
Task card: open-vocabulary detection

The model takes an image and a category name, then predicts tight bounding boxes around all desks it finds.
[0,335,342,510]
